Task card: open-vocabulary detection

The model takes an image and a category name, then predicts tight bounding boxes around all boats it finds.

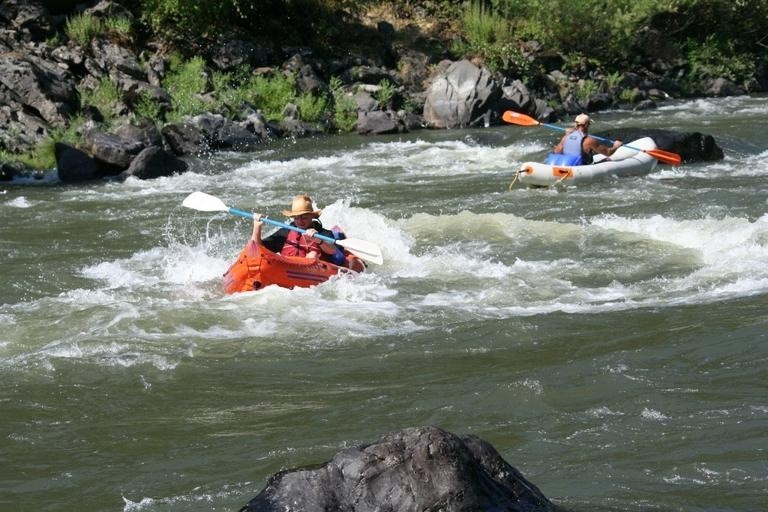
[220,218,368,296]
[518,136,660,187]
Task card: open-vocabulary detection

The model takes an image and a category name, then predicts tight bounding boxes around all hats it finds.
[575,113,593,125]
[282,195,321,220]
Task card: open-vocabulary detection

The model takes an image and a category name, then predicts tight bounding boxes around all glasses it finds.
[293,213,310,219]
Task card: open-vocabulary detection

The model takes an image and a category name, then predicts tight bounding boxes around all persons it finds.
[252,194,346,264]
[552,113,622,165]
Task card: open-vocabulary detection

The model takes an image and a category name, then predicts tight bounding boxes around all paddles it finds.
[502,111,682,166]
[183,191,384,264]
